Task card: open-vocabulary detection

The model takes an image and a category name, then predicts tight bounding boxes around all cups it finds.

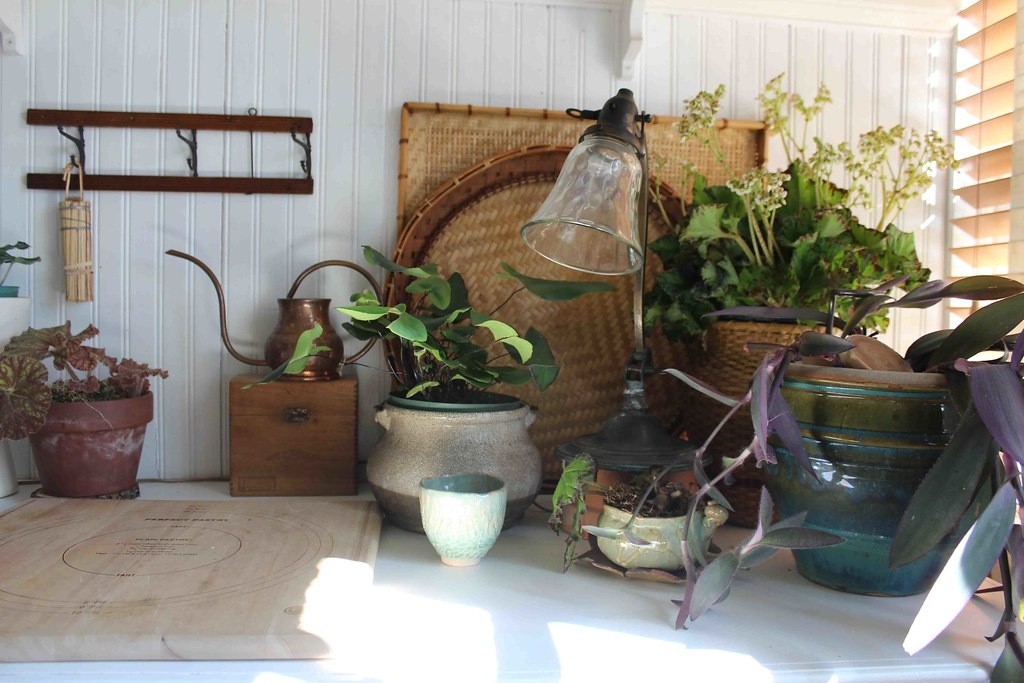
[417,472,507,567]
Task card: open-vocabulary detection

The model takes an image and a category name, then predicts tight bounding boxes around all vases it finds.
[685,322,835,527]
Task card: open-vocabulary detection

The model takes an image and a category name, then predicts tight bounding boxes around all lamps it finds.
[521,88,715,472]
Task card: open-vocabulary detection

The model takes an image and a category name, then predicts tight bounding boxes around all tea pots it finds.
[164,250,384,382]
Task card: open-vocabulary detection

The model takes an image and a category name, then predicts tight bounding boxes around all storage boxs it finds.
[228,366,358,497]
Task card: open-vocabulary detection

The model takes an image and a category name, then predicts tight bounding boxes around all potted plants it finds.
[0,241,41,297]
[546,455,726,572]
[601,272,1024,683]
[241,246,619,536]
[0,320,169,496]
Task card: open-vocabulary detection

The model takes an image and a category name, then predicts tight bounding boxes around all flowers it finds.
[645,73,961,326]
